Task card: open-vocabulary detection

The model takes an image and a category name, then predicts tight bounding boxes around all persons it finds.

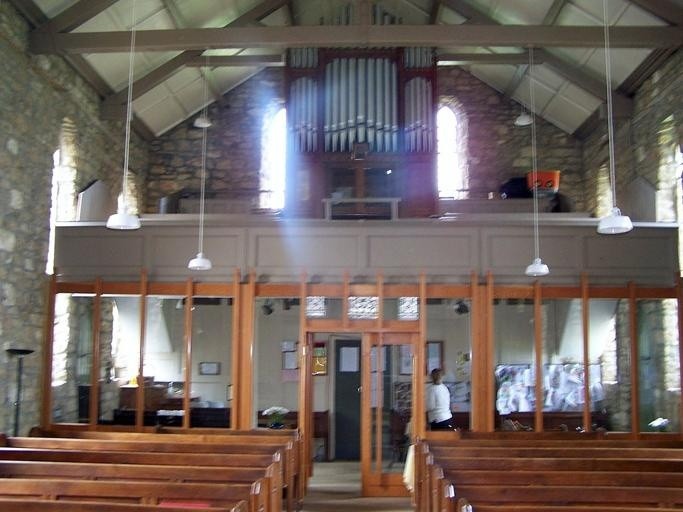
[423,368,453,431]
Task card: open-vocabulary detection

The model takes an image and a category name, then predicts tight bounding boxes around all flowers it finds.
[262,406,289,423]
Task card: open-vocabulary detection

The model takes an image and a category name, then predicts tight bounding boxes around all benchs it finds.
[411,432,683,511]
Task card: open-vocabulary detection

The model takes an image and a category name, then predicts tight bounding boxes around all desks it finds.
[120,383,169,411]
[257,409,329,462]
[321,196,402,221]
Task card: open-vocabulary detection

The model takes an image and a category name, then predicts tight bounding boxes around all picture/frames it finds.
[426,340,444,376]
[199,362,220,375]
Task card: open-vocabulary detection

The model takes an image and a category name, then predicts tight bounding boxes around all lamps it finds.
[194,74,211,128]
[514,65,533,127]
[524,45,549,276]
[106,0,141,231]
[596,0,633,234]
[188,45,211,270]
